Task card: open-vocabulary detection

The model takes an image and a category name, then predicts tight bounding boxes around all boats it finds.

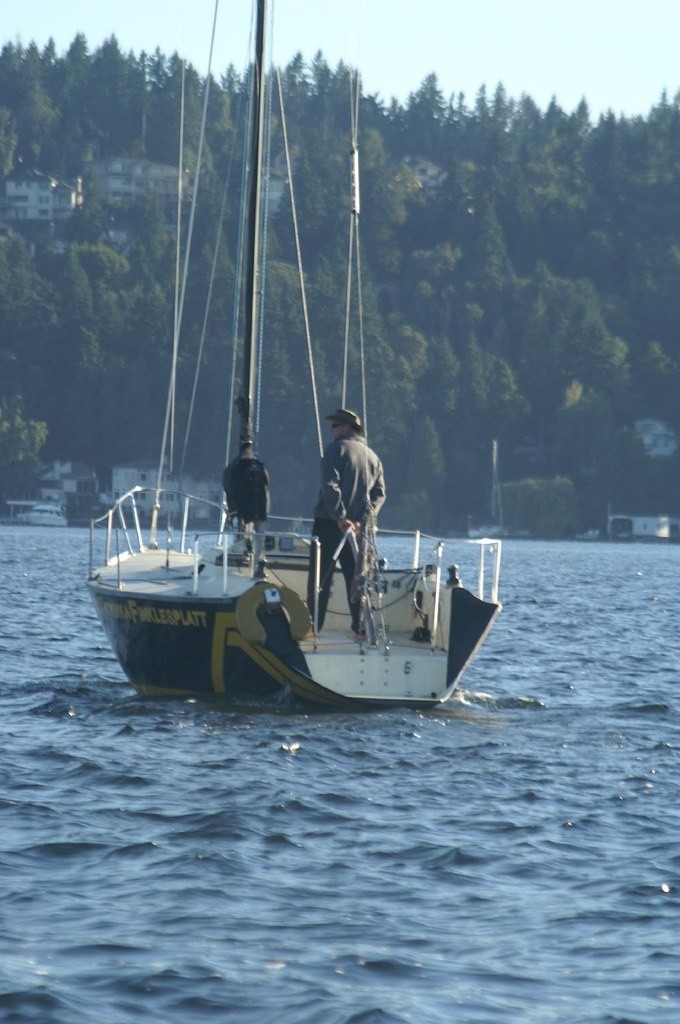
[18,504,68,527]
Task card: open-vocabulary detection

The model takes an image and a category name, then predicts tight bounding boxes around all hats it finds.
[324,408,363,431]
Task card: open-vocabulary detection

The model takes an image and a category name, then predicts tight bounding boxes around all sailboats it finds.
[83,0,503,715]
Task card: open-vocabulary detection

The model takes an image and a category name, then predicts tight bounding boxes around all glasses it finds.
[332,423,343,428]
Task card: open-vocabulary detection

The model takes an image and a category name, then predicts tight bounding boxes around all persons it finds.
[307,408,387,640]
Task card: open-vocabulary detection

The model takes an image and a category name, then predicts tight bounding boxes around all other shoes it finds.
[355,630,366,639]
